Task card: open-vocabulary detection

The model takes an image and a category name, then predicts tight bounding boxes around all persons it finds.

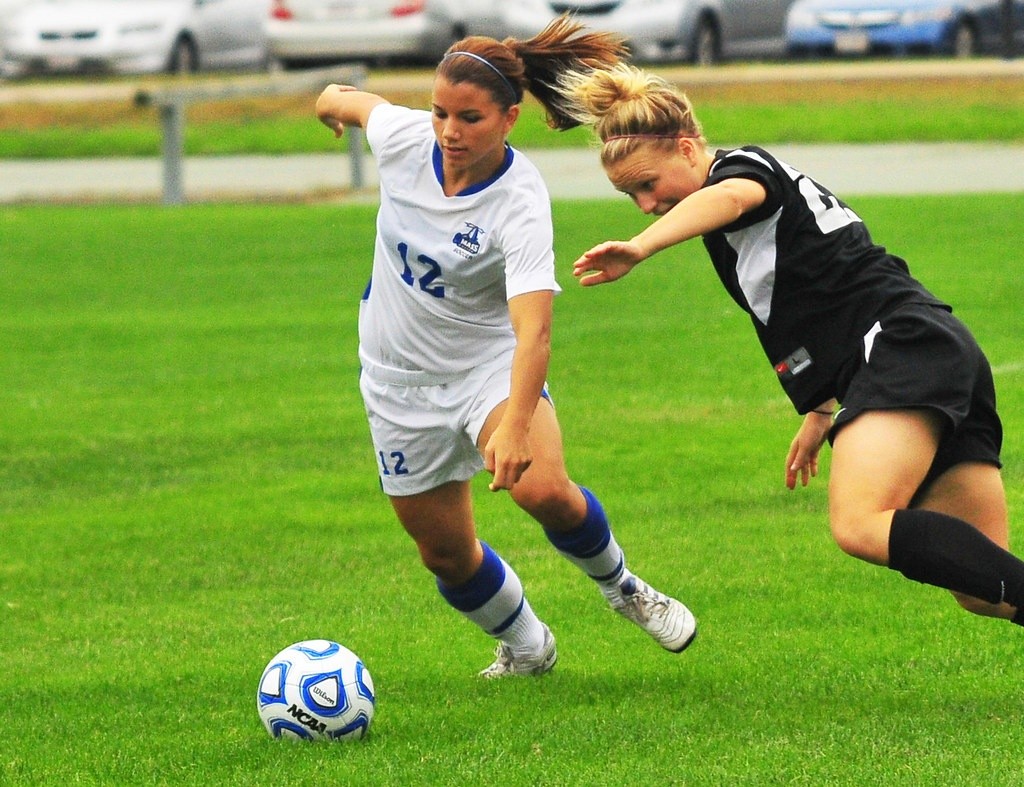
[315,9,700,682]
[542,57,1024,630]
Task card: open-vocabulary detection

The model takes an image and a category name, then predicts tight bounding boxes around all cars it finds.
[529,0,795,68]
[1,1,273,78]
[267,0,538,71]
[783,0,1024,62]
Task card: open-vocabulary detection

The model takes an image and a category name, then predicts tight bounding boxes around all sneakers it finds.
[610,575,698,652]
[474,622,557,679]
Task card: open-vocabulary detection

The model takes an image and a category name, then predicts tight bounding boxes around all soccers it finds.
[256,639,375,743]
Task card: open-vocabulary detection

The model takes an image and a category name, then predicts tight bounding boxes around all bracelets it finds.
[811,410,834,417]
